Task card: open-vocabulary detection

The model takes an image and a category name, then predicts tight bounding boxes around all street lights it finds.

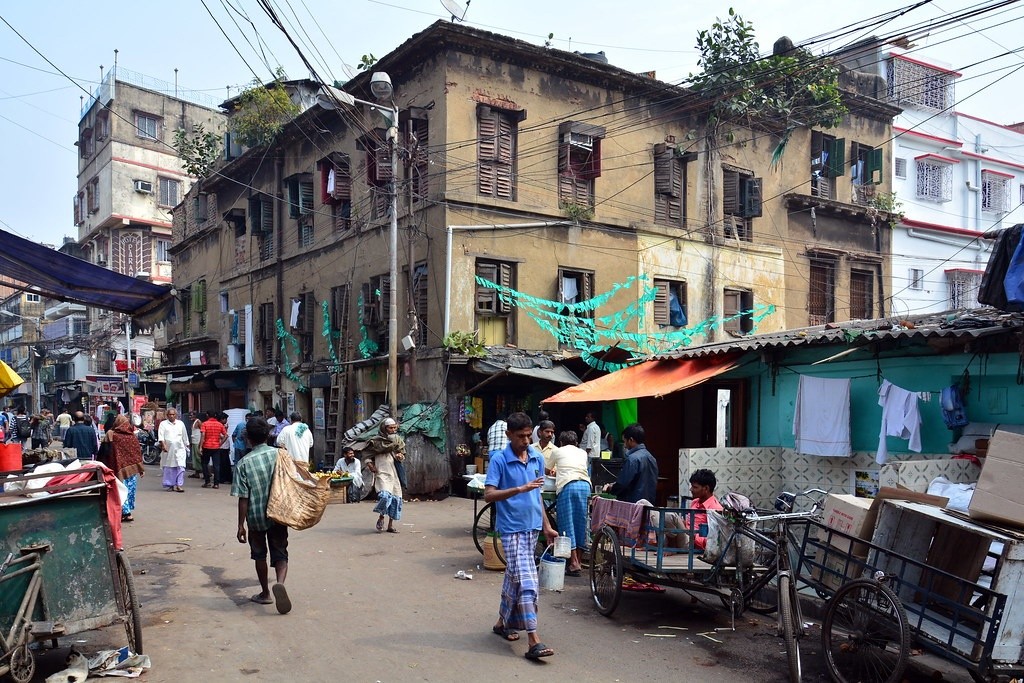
[315,71,400,425]
[0,310,40,414]
[126,272,155,416]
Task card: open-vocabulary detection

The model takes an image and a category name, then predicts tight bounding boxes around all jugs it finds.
[0,443,22,472]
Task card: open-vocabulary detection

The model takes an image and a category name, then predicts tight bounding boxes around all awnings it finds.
[541,351,760,403]
[466,355,583,390]
[0,228,178,338]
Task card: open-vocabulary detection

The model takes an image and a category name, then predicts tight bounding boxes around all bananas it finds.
[316,469,349,478]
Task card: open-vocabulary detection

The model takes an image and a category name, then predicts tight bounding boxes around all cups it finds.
[553,536,571,559]
[595,485,603,494]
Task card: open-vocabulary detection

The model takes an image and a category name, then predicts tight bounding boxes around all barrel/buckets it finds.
[483,532,507,570]
[538,553,566,590]
[466,465,477,474]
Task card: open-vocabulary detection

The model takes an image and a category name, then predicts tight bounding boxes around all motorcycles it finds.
[130,415,159,465]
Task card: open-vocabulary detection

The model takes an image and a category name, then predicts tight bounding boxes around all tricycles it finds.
[589,488,818,683]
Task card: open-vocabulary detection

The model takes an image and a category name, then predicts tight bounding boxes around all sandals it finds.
[202,483,211,487]
[122,514,134,521]
[213,485,219,489]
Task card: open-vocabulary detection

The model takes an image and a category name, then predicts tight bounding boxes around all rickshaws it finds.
[794,510,1024,683]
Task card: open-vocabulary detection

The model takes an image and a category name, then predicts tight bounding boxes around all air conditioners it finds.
[135,180,152,193]
[564,132,593,147]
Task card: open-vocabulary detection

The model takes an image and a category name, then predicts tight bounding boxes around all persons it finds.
[230,418,292,615]
[332,447,365,504]
[482,411,555,659]
[364,418,407,533]
[0,406,314,522]
[486,406,725,577]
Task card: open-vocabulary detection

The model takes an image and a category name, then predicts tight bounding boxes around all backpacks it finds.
[14,416,31,438]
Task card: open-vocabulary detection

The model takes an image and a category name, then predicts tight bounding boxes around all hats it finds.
[42,409,51,413]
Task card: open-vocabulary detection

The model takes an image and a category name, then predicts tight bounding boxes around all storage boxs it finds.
[813,428,1024,664]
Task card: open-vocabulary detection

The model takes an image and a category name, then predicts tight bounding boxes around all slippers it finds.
[566,566,581,576]
[493,625,520,641]
[525,644,554,658]
[176,487,184,492]
[386,528,400,533]
[376,519,384,530]
[251,592,272,603]
[189,473,200,478]
[272,583,292,614]
[168,487,175,491]
